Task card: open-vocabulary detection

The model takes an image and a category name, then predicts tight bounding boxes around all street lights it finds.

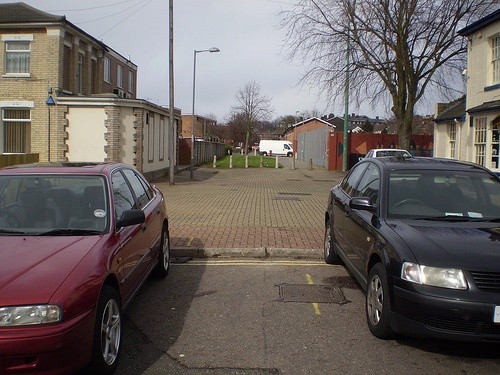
[189,47,221,179]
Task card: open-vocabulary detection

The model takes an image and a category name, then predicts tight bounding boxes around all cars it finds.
[357,148,412,162]
[322,157,500,357]
[0,163,169,374]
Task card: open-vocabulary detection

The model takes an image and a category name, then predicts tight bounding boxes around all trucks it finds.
[259,139,294,157]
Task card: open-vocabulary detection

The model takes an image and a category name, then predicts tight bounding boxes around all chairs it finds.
[10,186,104,227]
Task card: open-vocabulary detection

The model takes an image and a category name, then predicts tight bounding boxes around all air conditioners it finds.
[112,88,129,99]
